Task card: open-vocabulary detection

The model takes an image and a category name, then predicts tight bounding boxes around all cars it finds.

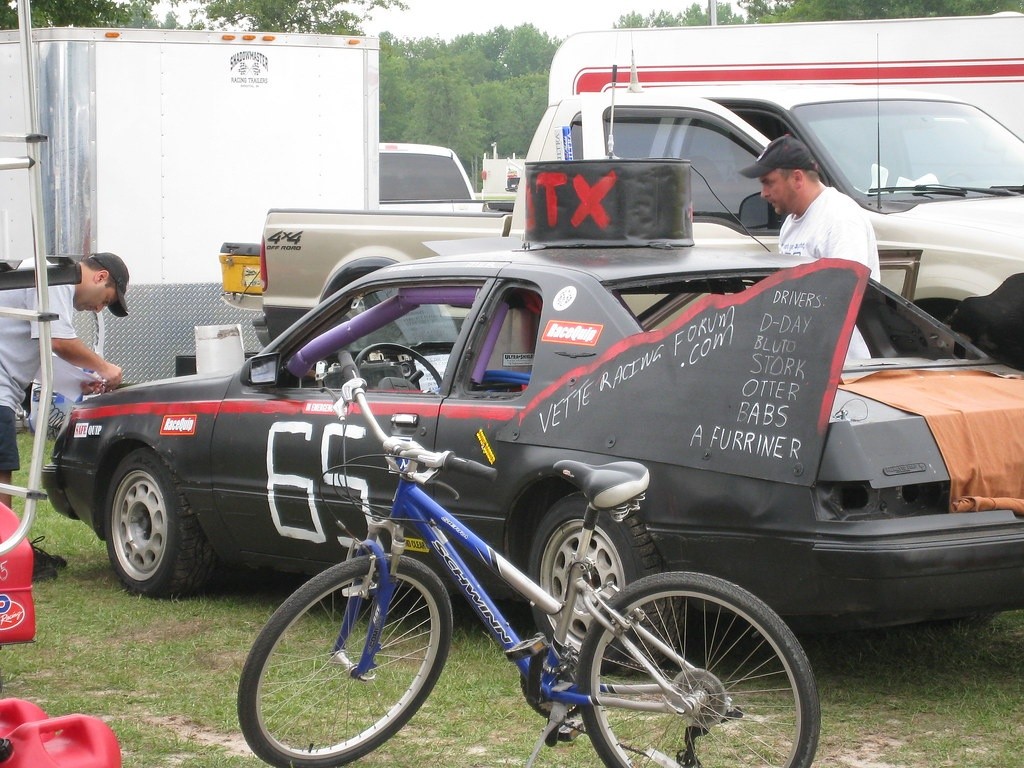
[41,158,1024,647]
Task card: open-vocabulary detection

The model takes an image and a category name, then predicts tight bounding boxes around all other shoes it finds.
[31,563,58,584]
[29,536,67,569]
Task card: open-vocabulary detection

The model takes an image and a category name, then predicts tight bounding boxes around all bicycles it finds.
[229,361,819,767]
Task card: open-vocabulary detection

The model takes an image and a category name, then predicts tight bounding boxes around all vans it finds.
[380,144,486,217]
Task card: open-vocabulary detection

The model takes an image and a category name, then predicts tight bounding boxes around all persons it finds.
[0,253,130,580]
[742,135,880,360]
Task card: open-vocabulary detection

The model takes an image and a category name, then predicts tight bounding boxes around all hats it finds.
[88,252,130,317]
[739,133,818,178]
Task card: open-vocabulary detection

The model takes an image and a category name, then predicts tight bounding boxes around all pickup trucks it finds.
[231,89,1023,359]
